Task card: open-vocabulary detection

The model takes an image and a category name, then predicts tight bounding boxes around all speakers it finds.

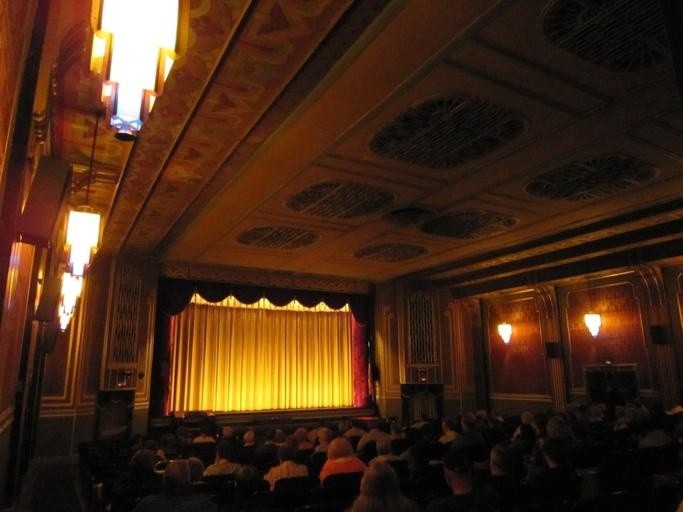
[18,155,73,248]
[649,324,670,345]
[36,287,60,321]
[545,342,563,358]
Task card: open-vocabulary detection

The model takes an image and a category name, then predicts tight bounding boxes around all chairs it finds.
[3,402,682,505]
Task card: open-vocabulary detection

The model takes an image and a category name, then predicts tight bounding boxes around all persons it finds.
[110,397,683,512]
